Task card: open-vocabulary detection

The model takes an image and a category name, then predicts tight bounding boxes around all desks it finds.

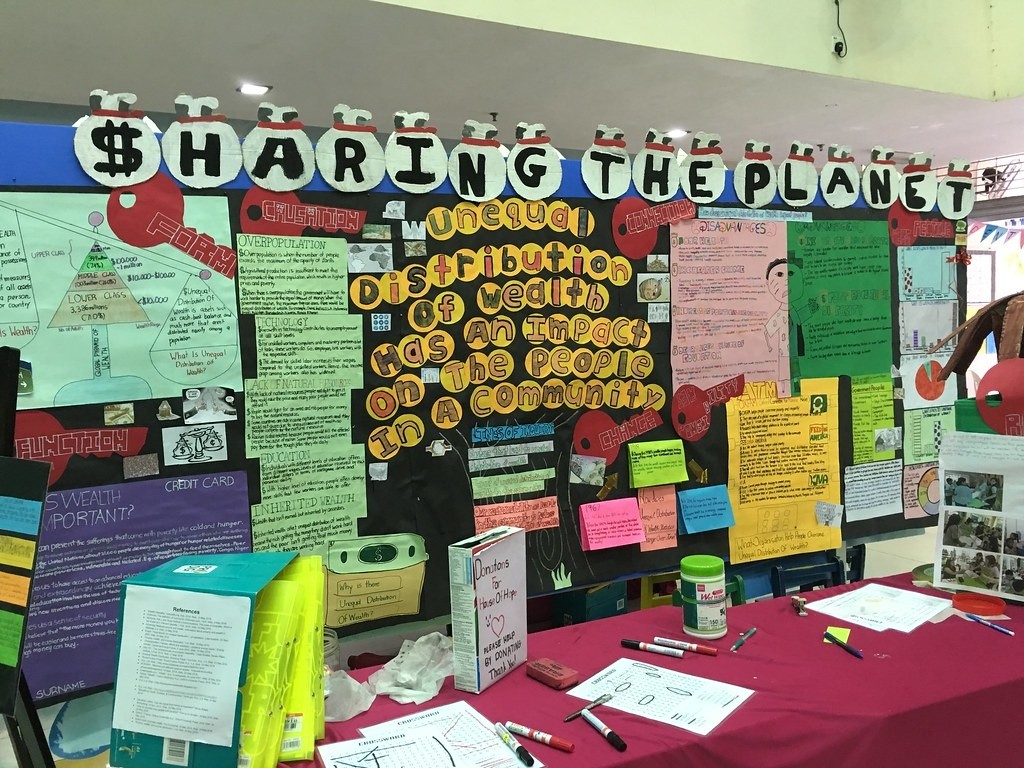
[275,573,1024,768]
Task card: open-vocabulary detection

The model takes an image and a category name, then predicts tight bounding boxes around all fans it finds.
[982,158,1022,200]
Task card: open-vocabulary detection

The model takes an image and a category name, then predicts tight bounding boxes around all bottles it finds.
[322,628,340,717]
[680,554,728,641]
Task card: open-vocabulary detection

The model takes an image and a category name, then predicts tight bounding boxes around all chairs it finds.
[640,556,845,613]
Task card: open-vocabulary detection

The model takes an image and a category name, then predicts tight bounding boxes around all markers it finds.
[620,637,685,659]
[653,636,719,657]
[581,709,628,752]
[495,722,535,767]
[504,721,576,753]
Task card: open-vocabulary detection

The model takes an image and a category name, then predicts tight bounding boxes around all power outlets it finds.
[831,36,845,54]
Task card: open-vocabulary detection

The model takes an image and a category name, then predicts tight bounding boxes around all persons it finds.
[959,517,1024,554]
[983,478,999,508]
[954,476,972,506]
[972,474,988,502]
[981,555,1000,590]
[943,514,966,547]
[945,478,955,505]
[970,552,985,575]
[1003,567,1024,596]
[943,558,966,580]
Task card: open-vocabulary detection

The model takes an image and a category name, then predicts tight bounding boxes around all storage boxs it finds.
[447,524,528,694]
[110,551,301,768]
[550,580,627,628]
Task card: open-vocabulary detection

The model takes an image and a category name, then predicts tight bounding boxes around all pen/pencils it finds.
[964,613,1017,637]
[563,692,612,723]
[728,627,758,652]
[823,630,865,660]
